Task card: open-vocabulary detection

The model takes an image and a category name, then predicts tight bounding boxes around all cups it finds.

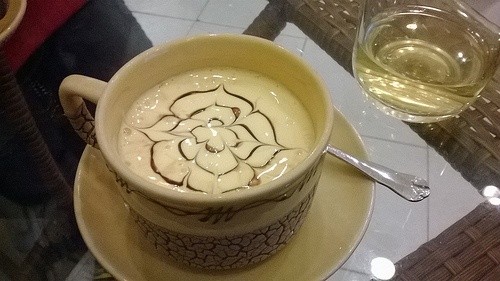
[59,33,335,268]
[352,0,500,123]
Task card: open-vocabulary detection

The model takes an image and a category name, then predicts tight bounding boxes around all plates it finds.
[72,102,376,281]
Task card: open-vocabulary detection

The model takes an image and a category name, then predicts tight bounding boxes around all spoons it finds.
[327,143,430,203]
